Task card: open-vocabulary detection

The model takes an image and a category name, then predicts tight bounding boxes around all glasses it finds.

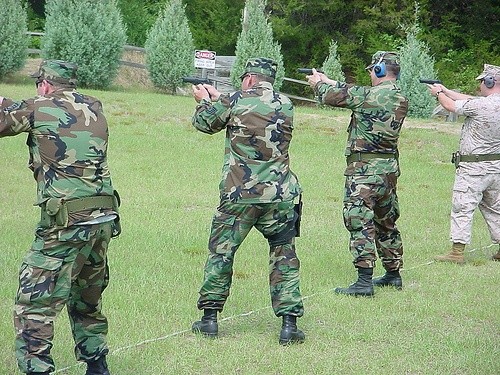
[35,79,53,88]
[241,73,257,81]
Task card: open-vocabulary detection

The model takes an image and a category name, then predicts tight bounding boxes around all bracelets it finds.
[436,91,443,101]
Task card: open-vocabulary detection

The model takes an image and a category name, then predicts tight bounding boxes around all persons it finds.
[0,58,122,375]
[426,63,500,264]
[189,57,304,347]
[306,50,409,296]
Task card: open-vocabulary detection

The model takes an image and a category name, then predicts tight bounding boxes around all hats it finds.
[238,58,279,79]
[365,51,400,73]
[30,60,78,84]
[475,64,500,79]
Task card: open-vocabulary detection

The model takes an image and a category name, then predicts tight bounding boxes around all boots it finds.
[372,271,402,287]
[435,243,465,264]
[490,243,500,261]
[85,355,110,375]
[192,309,218,336]
[334,268,374,295]
[279,315,305,346]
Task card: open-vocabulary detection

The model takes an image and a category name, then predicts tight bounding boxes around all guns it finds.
[183,77,213,99]
[419,78,441,85]
[297,67,324,75]
